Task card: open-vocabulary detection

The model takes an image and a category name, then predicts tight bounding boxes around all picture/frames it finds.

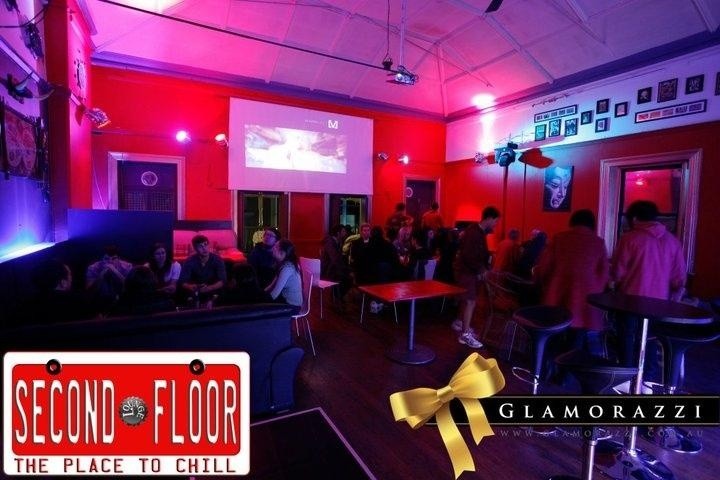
[534,71,720,141]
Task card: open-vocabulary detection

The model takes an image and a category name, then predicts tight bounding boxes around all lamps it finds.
[88,106,110,127]
[394,1,419,84]
[378,152,388,161]
[214,134,228,148]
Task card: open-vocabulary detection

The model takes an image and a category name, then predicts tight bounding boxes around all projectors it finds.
[386,73,415,85]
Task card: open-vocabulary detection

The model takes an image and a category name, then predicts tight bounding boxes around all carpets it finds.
[178,406,375,480]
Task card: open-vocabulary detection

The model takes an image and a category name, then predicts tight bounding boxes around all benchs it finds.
[0,237,268,419]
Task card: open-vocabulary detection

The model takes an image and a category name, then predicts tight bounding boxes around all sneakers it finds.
[458,333,483,348]
[615,380,654,394]
[451,320,477,337]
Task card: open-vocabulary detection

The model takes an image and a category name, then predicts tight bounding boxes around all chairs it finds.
[173,226,720,480]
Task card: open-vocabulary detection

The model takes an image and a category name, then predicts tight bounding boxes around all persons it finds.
[612,199,688,302]
[532,207,611,332]
[544,165,573,209]
[320,199,548,348]
[19,227,303,319]
